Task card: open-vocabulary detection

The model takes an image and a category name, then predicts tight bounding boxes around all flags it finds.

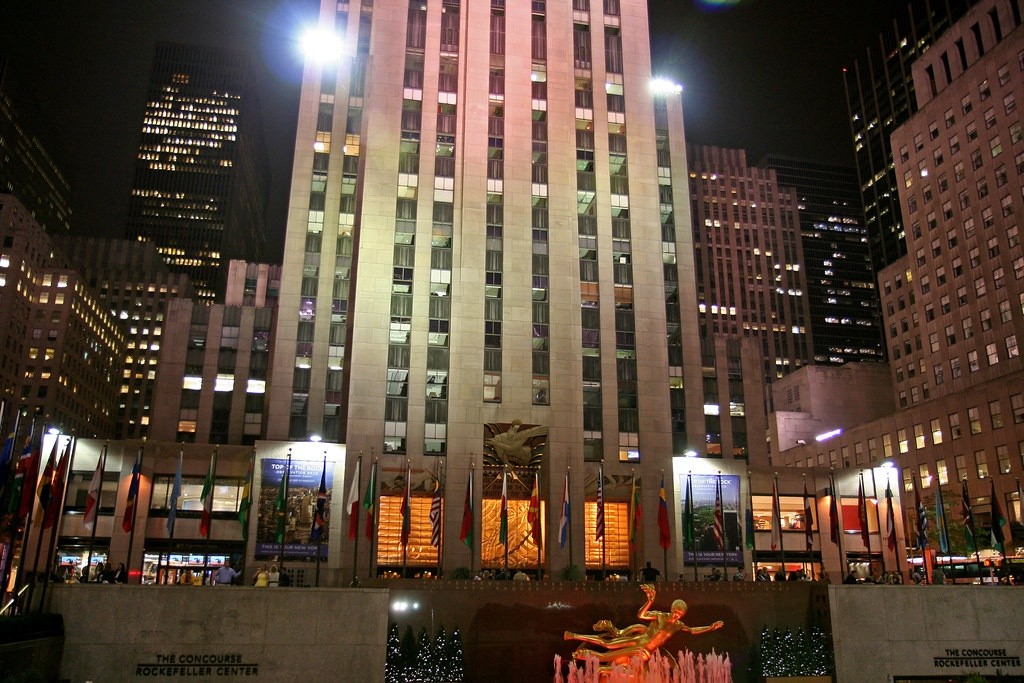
[0,430,106,541]
[122,446,1007,553]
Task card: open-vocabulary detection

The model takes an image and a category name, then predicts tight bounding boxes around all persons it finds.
[641,561,664,581]
[707,566,810,581]
[251,565,291,588]
[212,561,241,587]
[563,583,724,676]
[472,567,532,592]
[53,561,129,585]
[843,567,926,585]
[990,561,996,585]
